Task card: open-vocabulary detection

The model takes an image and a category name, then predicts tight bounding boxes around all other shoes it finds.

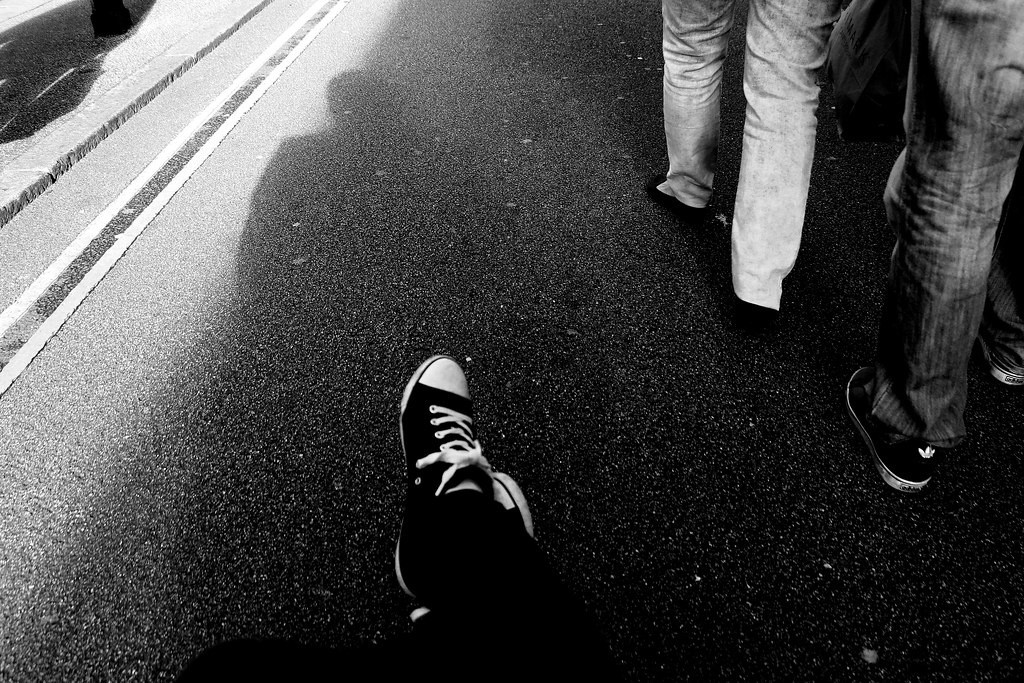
[646,174,708,223]
[977,330,1024,385]
[847,366,938,492]
[735,292,779,329]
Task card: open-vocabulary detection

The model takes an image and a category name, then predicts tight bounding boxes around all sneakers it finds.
[394,355,534,637]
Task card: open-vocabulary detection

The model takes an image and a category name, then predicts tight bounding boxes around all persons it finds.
[169,354,629,683]
[647,0,1024,493]
